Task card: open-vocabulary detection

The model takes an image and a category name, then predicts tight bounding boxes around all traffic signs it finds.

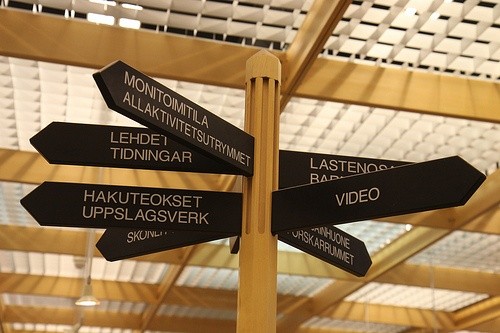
[20,60,487,278]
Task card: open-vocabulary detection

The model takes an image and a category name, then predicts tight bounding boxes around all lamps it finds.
[74,276,103,306]
